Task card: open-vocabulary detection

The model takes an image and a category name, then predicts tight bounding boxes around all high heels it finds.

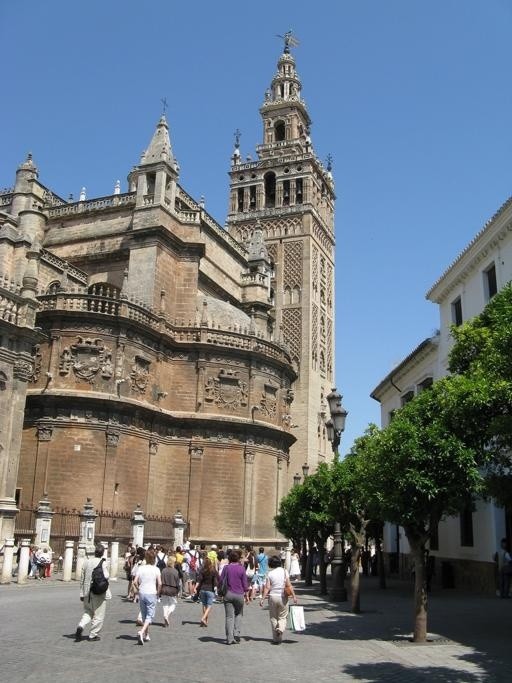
[272,627,286,643]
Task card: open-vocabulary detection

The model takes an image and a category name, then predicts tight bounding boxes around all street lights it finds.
[325,388,348,601]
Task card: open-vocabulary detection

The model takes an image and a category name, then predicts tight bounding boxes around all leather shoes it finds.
[75,625,84,639]
[227,635,242,645]
[87,635,101,642]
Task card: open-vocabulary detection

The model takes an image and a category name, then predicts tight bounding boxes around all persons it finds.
[58,554,63,569]
[410,549,437,592]
[121,542,302,645]
[12,543,52,581]
[75,544,112,642]
[492,538,512,598]
[311,545,381,575]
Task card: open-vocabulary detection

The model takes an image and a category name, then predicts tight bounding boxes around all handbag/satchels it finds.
[289,605,306,632]
[217,578,229,597]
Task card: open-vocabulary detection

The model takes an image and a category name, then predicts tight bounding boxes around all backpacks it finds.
[155,553,166,572]
[88,558,109,595]
[187,550,198,572]
